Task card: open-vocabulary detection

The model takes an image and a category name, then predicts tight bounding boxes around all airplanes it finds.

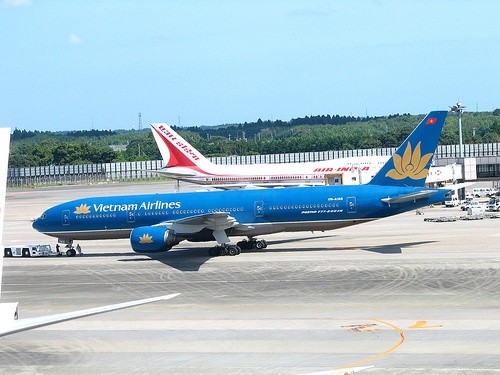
[148,123,392,190]
[32,111,477,257]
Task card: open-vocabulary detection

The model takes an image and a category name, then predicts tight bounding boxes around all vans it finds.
[445,186,500,211]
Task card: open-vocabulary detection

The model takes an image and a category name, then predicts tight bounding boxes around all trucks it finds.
[432,158,477,184]
